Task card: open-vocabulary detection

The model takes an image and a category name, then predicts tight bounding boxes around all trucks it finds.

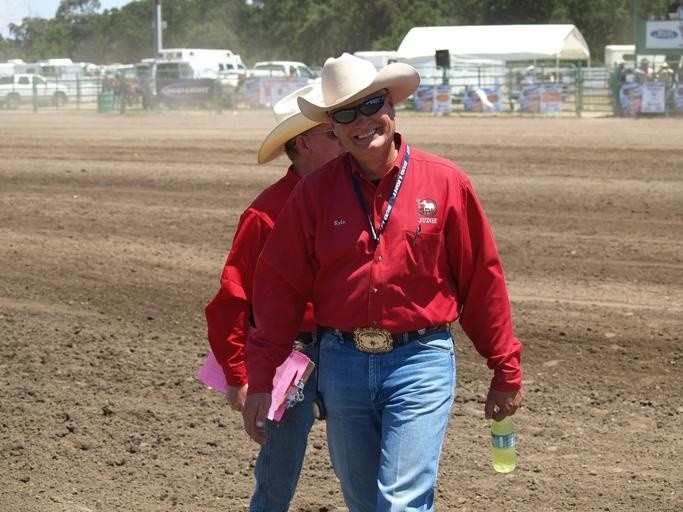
[160,46,248,80]
[219,61,316,105]
[9,56,141,103]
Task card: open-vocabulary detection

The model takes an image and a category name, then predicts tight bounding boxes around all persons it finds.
[239,47,527,512]
[605,55,678,120]
[201,77,350,511]
[98,69,151,112]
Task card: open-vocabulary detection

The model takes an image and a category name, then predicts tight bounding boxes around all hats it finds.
[297,52,421,124]
[257,82,326,164]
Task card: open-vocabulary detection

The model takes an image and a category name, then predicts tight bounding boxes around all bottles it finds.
[490,406,517,474]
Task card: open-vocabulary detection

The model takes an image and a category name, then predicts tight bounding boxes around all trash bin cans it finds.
[98,94,113,114]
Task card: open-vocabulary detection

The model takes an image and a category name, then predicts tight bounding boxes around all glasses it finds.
[304,129,338,139]
[332,93,388,125]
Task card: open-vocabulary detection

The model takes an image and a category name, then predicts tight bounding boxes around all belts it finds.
[325,326,447,354]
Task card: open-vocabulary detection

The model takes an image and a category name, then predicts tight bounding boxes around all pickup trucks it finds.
[0,73,71,111]
